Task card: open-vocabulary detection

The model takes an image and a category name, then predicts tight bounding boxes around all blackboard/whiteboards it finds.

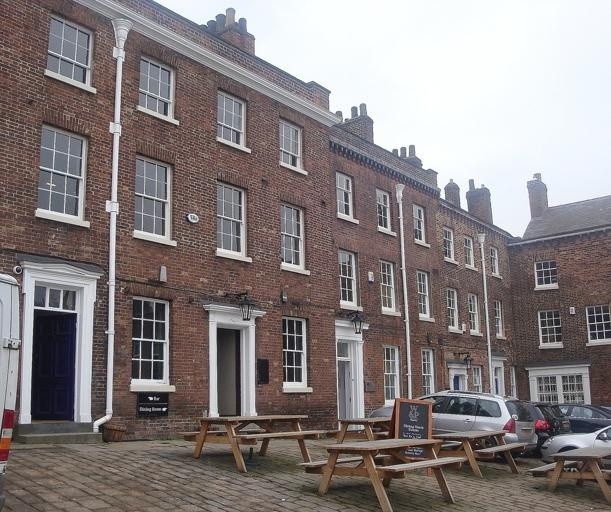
[395,398,433,461]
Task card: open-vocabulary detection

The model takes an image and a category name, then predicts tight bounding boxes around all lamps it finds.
[226,292,255,322]
[346,310,364,334]
[453,352,474,370]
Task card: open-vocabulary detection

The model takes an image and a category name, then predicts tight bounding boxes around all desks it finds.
[189,413,311,472]
[548,447,611,505]
[316,417,510,510]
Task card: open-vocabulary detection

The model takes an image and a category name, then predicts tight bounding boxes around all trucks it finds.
[0,275,21,512]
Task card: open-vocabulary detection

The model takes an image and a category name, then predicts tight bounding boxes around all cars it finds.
[370,390,610,472]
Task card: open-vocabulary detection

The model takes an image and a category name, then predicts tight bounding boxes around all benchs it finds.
[176,426,339,475]
[295,442,530,512]
[526,461,577,478]
